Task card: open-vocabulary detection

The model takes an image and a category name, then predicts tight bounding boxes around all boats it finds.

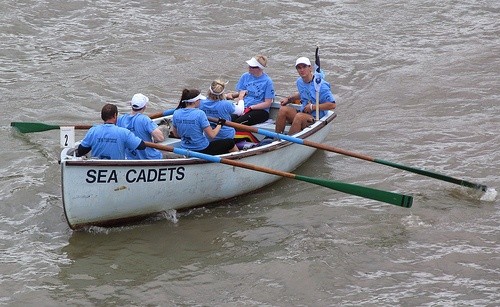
[58,97,339,232]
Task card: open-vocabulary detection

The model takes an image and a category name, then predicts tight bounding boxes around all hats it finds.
[295,57,311,67]
[245,57,265,70]
[182,92,207,103]
[131,93,149,109]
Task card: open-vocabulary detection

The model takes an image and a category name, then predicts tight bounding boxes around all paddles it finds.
[140,140,414,208]
[208,116,489,191]
[10,122,92,133]
[147,91,248,119]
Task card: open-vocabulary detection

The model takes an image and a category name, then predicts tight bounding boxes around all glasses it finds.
[249,65,259,69]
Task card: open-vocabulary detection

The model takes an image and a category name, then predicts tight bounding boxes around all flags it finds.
[314,47,324,92]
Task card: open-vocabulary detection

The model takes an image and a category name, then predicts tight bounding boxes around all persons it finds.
[199,79,260,143]
[275,57,336,135]
[115,93,185,159]
[172,88,240,156]
[226,55,275,131]
[78,103,146,160]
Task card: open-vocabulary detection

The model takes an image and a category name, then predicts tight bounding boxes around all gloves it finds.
[241,107,253,116]
[217,117,226,128]
